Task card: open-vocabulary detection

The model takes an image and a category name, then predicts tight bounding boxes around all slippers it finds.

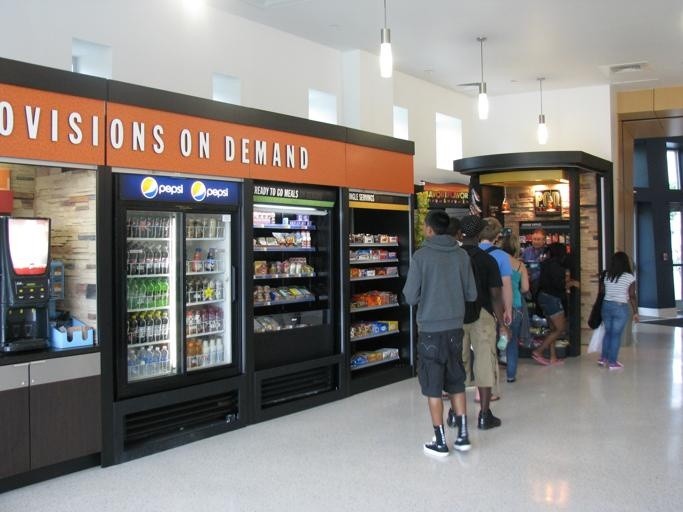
[441,394,450,401]
[550,359,565,366]
[474,394,499,403]
[530,355,547,365]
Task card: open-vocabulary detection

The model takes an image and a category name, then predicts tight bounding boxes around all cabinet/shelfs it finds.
[253,202,336,371]
[0,353,103,480]
[350,208,403,373]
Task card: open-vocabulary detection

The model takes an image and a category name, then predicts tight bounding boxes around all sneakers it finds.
[452,440,470,452]
[506,376,516,382]
[596,357,607,367]
[422,441,449,457]
[608,361,624,370]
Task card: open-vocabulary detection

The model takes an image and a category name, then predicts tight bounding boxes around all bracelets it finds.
[633,309,638,314]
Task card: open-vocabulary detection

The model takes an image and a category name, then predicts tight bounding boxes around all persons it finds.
[496,234,530,383]
[446,215,514,431]
[401,210,478,458]
[440,218,462,400]
[521,227,547,279]
[532,242,580,366]
[596,251,640,372]
[478,217,513,404]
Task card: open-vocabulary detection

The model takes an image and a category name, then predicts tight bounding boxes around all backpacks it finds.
[464,248,487,324]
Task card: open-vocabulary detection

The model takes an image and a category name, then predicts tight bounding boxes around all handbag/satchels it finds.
[586,293,604,330]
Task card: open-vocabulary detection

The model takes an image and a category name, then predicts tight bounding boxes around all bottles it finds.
[302,233,306,247]
[126,277,168,309]
[186,305,223,335]
[307,233,312,249]
[290,263,295,275]
[498,330,509,351]
[186,334,224,368]
[127,244,168,275]
[127,214,167,238]
[186,277,224,303]
[206,247,215,271]
[296,263,301,273]
[428,191,468,204]
[187,217,224,238]
[128,344,168,379]
[126,310,169,344]
[192,248,202,271]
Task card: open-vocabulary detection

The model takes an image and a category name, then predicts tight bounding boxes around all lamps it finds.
[476,36,488,120]
[379,0,393,78]
[536,77,546,145]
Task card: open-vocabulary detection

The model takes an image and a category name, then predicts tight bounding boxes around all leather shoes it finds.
[446,409,456,429]
[477,408,500,430]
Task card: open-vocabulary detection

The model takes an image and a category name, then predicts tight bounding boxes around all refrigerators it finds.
[113,171,244,466]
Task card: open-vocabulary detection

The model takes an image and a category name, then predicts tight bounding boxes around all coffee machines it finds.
[0,215,50,352]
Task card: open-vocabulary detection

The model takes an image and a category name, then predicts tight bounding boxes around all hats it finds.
[460,216,486,236]
